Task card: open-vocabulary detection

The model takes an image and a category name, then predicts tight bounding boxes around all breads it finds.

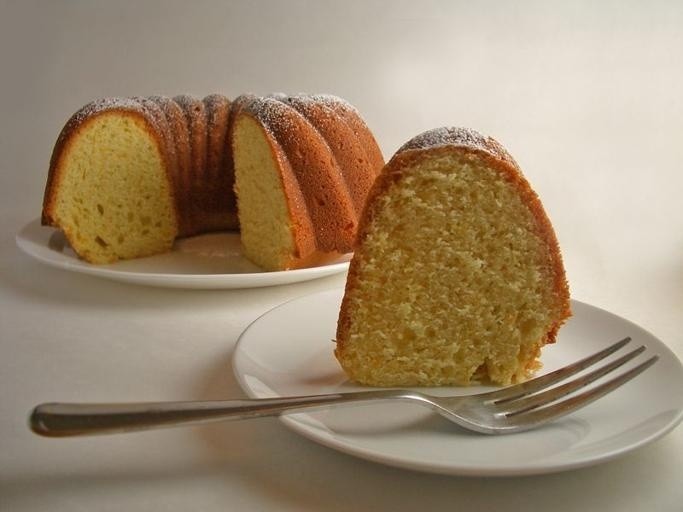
[41,93,386,273]
[332,126,573,389]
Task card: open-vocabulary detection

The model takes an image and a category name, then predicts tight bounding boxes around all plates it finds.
[11,213,352,291]
[229,284,683,478]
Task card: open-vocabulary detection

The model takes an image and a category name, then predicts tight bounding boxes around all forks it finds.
[27,336,660,438]
